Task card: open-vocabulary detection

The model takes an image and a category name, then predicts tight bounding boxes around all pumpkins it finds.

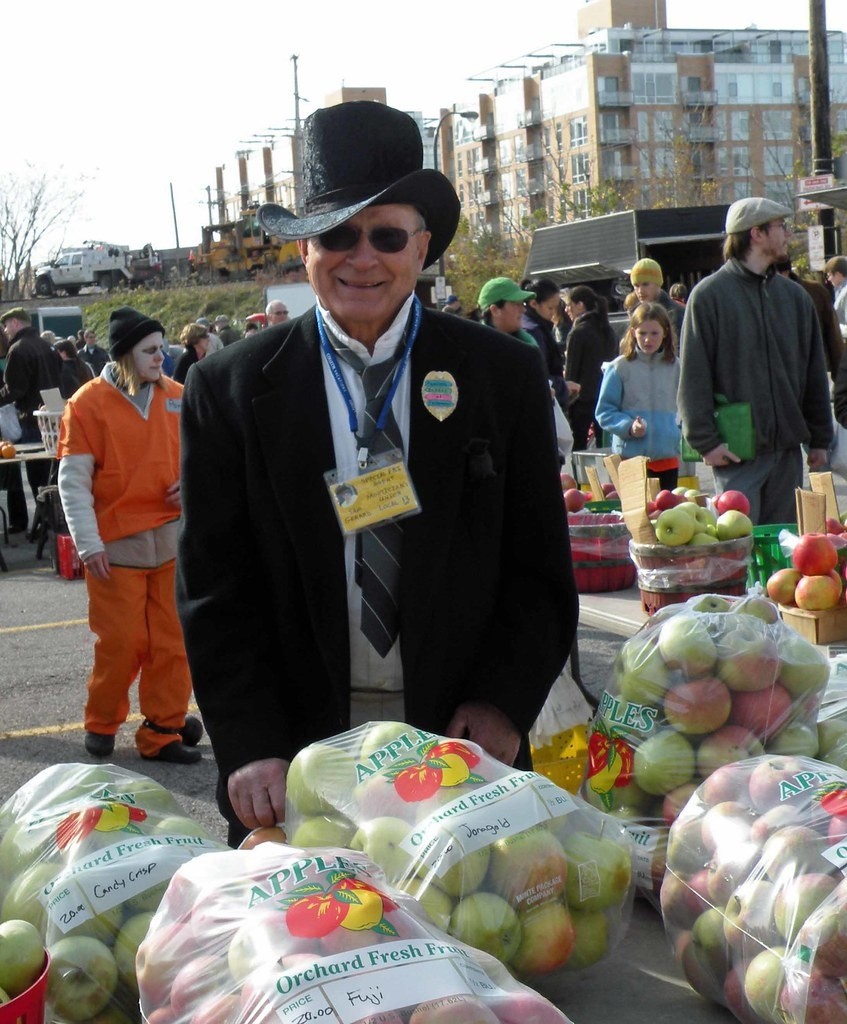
[0,437,15,458]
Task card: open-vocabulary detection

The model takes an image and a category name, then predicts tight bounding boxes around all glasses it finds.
[770,221,788,231]
[200,335,209,339]
[275,310,289,315]
[318,221,424,253]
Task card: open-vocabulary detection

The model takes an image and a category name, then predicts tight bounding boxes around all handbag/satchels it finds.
[680,394,756,461]
[549,379,574,457]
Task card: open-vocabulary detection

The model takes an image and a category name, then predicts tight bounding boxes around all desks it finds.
[0,451,56,558]
[571,584,645,708]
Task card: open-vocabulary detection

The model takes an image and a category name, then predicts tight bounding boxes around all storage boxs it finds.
[777,601,847,644]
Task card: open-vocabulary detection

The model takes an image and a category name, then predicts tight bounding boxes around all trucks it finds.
[34,239,164,296]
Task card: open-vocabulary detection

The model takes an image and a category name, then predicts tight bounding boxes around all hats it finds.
[477,278,537,310]
[630,258,664,288]
[108,307,165,360]
[0,307,31,322]
[725,197,793,234]
[256,100,461,273]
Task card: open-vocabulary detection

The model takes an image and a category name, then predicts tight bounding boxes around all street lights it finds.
[432,110,479,312]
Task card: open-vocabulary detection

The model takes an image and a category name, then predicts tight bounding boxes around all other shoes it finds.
[8,526,27,533]
[85,732,115,756]
[140,740,202,764]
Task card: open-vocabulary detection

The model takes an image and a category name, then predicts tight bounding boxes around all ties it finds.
[318,307,414,658]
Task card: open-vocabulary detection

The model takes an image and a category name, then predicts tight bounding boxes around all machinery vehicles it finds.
[189,201,302,278]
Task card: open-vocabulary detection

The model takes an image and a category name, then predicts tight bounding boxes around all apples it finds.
[581,593,847,1024]
[765,512,847,610]
[647,486,752,546]
[0,721,630,1024]
[600,482,617,499]
[560,473,585,512]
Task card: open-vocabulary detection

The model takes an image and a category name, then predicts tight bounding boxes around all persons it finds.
[519,278,581,411]
[775,255,847,481]
[624,258,688,357]
[595,302,681,491]
[549,287,619,492]
[676,196,835,524]
[442,295,459,314]
[0,307,111,540]
[176,101,580,848]
[56,307,201,764]
[160,300,288,384]
[340,487,357,508]
[461,276,536,344]
